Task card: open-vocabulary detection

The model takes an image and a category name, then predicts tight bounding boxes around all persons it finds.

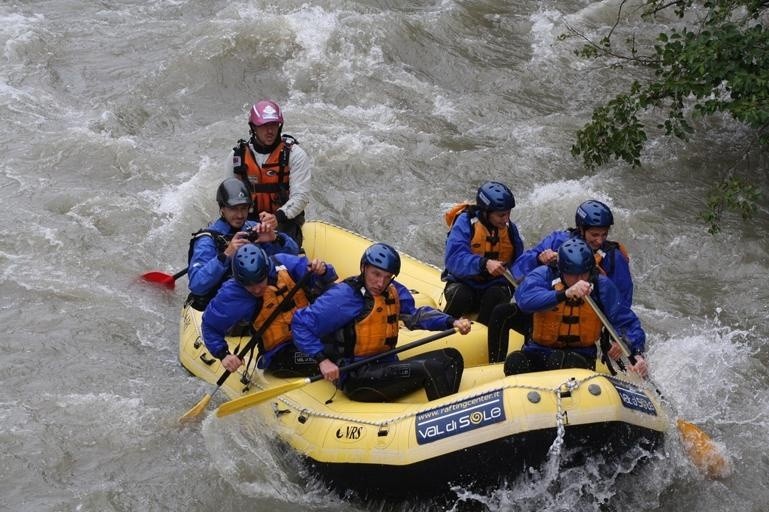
[436,180,526,327]
[198,243,339,381]
[182,179,301,309]
[500,238,650,383]
[284,241,472,402]
[223,97,311,250]
[504,198,635,363]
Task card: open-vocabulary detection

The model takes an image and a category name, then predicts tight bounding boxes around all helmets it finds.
[361,242,401,276]
[216,177,252,209]
[248,99,284,128]
[231,242,270,283]
[559,236,594,275]
[574,199,615,229]
[476,180,515,212]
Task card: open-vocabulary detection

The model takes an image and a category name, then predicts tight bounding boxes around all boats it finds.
[173,215,674,504]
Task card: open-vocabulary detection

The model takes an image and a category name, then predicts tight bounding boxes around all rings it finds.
[329,376,333,381]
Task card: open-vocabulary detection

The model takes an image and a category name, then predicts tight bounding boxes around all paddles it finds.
[585,293,725,476]
[141,266,190,290]
[217,327,461,417]
[179,270,312,422]
